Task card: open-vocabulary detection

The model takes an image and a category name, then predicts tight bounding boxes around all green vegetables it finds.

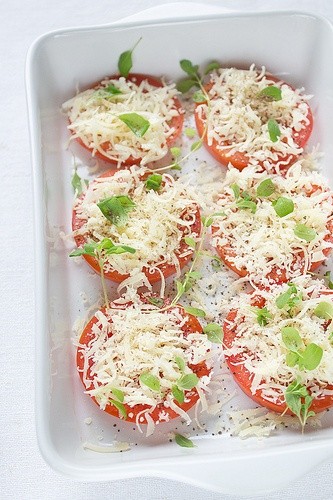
[71,37,333,448]
